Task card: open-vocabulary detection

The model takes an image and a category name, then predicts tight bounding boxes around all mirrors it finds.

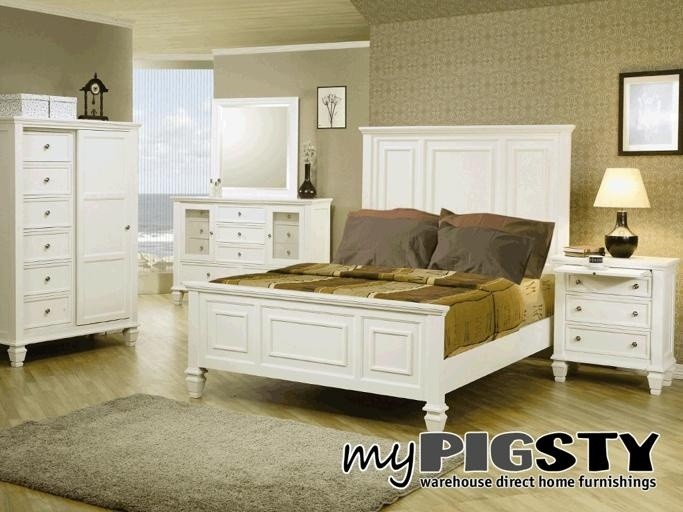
[209,94,301,199]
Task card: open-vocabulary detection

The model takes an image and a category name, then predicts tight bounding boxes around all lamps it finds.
[591,164,652,260]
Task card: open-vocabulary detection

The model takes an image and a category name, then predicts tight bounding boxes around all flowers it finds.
[300,140,316,163]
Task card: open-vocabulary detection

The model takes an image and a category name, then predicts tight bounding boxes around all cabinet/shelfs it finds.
[168,195,333,306]
[0,113,140,368]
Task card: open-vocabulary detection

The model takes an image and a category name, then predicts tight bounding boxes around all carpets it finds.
[2,392,485,511]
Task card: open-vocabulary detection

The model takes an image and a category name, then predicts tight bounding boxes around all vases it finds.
[297,163,316,199]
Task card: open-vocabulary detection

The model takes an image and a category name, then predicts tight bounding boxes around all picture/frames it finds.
[315,84,347,130]
[615,68,682,158]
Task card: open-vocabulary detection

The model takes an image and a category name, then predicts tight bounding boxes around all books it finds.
[563,251,605,258]
[564,246,605,254]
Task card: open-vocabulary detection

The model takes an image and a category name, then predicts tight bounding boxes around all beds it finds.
[178,258,557,434]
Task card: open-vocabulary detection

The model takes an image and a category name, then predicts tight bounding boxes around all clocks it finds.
[76,71,108,122]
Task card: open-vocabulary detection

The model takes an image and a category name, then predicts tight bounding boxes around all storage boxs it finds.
[1,92,79,122]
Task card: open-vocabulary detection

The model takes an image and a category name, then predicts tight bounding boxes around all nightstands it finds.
[548,249,680,396]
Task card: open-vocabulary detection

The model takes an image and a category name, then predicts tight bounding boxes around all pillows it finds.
[435,208,557,280]
[426,220,535,288]
[327,214,440,269]
[346,206,440,224]
